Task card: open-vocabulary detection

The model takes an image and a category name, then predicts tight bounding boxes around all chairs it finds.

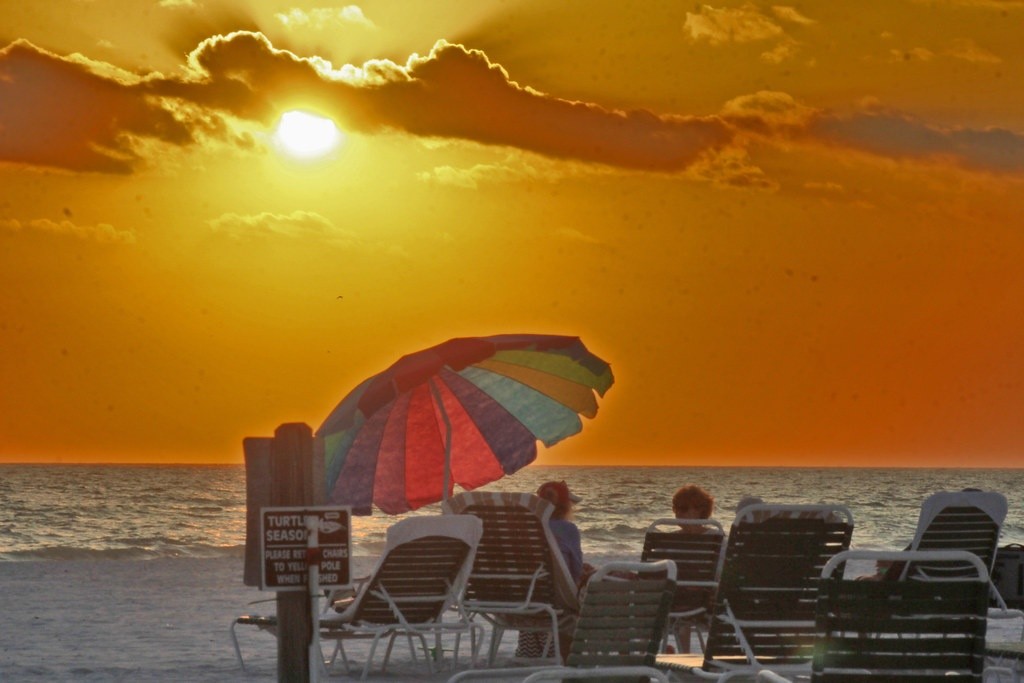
[228,490,1024,683]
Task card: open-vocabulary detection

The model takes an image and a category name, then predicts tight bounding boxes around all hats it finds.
[537,480,583,504]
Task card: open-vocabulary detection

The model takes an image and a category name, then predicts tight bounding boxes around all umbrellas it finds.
[316,335,615,516]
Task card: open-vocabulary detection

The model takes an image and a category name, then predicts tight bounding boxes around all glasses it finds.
[672,506,697,513]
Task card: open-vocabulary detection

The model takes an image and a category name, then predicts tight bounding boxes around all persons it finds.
[671,485,721,653]
[854,487,983,581]
[535,479,595,585]
